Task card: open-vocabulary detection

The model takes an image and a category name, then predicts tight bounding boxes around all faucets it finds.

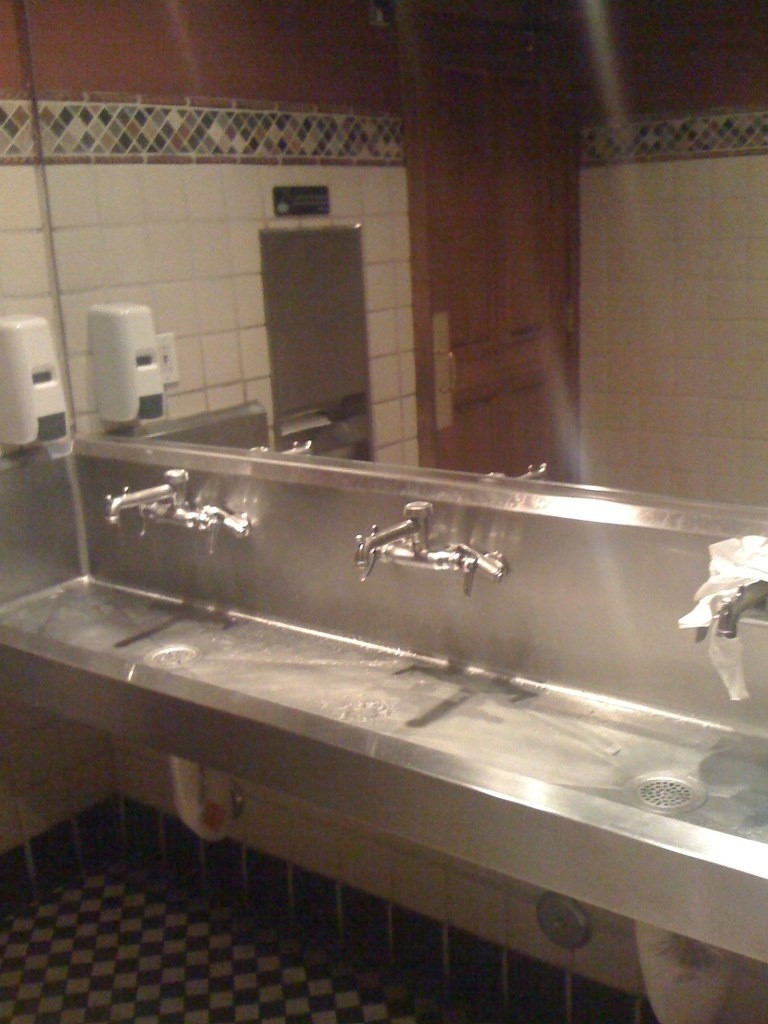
[248,438,318,456]
[484,460,548,481]
[103,467,251,540]
[352,501,510,585]
[714,578,768,641]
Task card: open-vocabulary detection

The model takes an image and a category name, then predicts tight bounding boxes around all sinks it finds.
[0,574,768,965]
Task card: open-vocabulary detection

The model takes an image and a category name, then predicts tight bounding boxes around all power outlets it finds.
[158,331,181,385]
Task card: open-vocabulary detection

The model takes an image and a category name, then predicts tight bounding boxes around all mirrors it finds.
[16,0,768,518]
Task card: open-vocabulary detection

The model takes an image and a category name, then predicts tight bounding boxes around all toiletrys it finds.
[86,300,169,434]
[0,312,77,464]
